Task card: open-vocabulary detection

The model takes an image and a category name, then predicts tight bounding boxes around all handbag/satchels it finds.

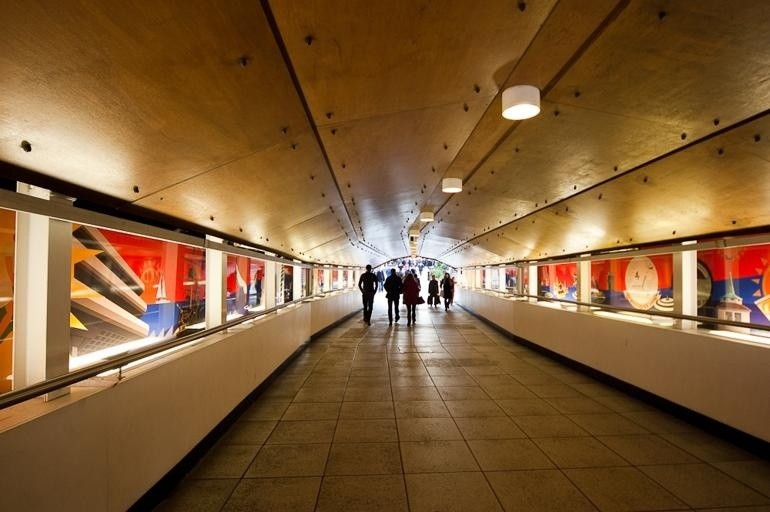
[415,295,425,304]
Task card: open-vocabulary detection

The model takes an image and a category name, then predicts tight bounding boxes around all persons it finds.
[402,273,420,327]
[374,256,435,292]
[358,264,378,326]
[383,268,403,326]
[450,276,458,306]
[428,274,439,307]
[440,272,454,311]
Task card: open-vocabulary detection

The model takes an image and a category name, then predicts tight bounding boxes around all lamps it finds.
[501,84,541,120]
[441,177,462,193]
[420,212,434,221]
